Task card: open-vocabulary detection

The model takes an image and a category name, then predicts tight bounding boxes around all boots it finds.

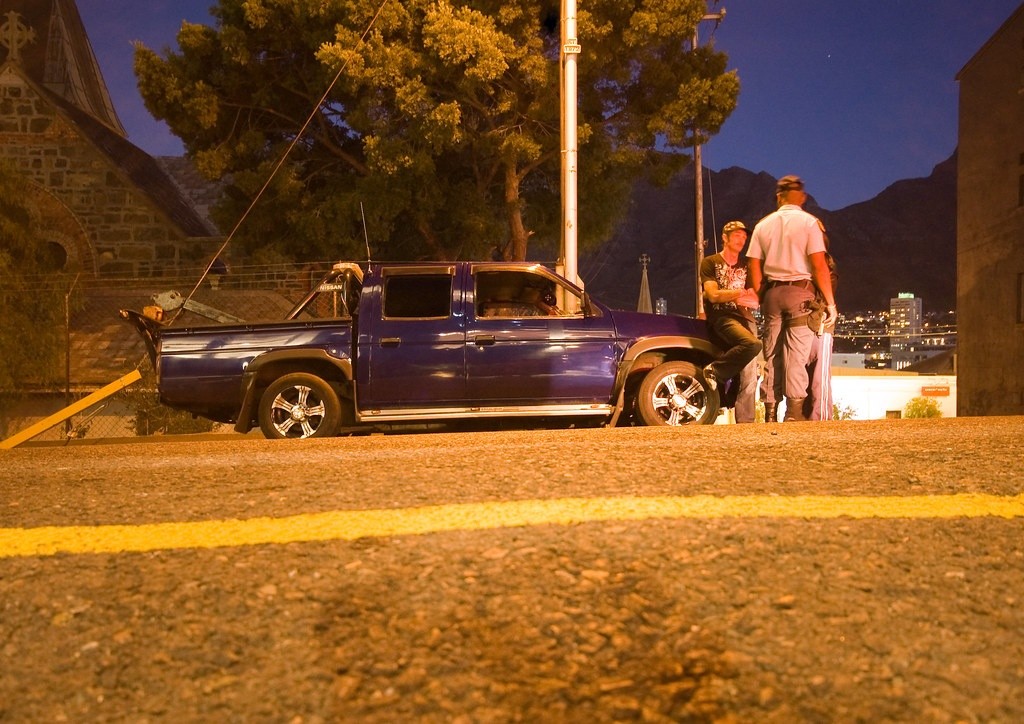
[765,402,778,422]
[783,398,809,422]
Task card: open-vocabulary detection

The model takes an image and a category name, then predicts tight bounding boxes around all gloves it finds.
[823,304,838,328]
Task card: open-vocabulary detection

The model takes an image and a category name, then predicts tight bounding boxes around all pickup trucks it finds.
[155,260,739,439]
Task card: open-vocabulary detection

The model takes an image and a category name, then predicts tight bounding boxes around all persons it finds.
[802,234,835,421]
[745,175,838,423]
[700,221,763,424]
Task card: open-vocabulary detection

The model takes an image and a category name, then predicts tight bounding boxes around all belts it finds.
[770,280,815,293]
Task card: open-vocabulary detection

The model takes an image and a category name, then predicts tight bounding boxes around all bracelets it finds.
[827,304,836,308]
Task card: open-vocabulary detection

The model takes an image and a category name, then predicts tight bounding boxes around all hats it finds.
[723,221,751,235]
[775,175,803,194]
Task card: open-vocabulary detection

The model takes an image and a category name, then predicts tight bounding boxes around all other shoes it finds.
[703,363,718,391]
